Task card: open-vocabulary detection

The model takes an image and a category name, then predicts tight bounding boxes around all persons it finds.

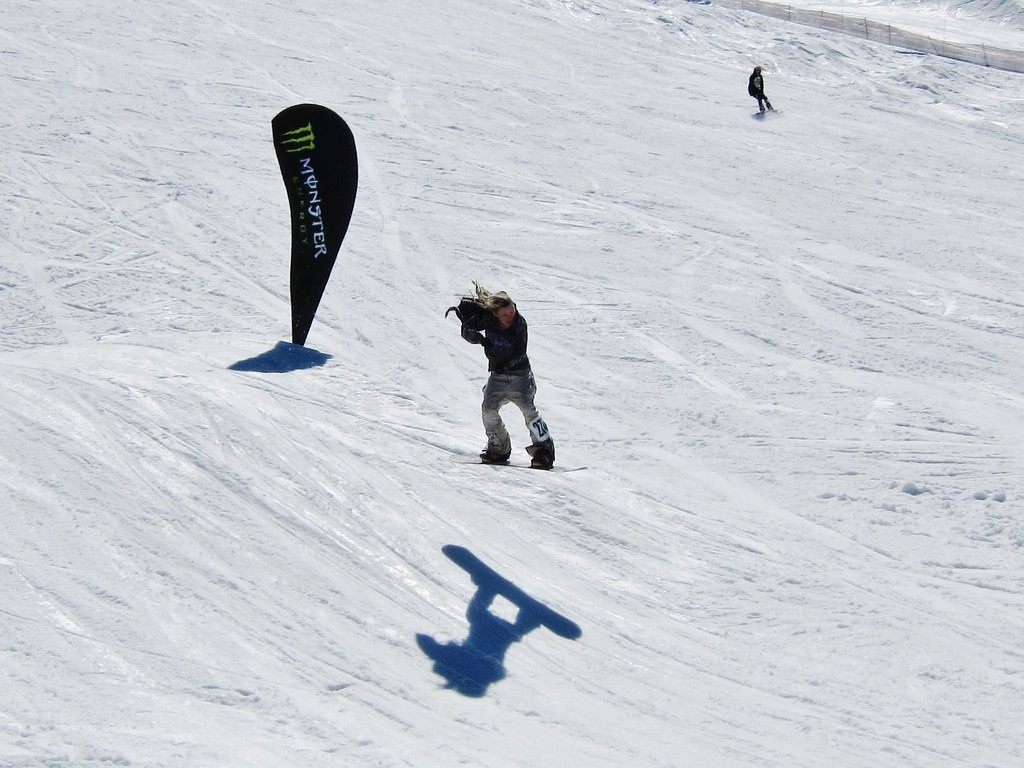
[747,66,774,112]
[455,281,555,470]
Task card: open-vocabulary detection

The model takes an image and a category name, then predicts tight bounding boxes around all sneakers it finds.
[531,448,553,468]
[479,448,511,463]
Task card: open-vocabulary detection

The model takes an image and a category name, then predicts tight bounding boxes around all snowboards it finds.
[451,460,587,474]
[753,109,784,117]
